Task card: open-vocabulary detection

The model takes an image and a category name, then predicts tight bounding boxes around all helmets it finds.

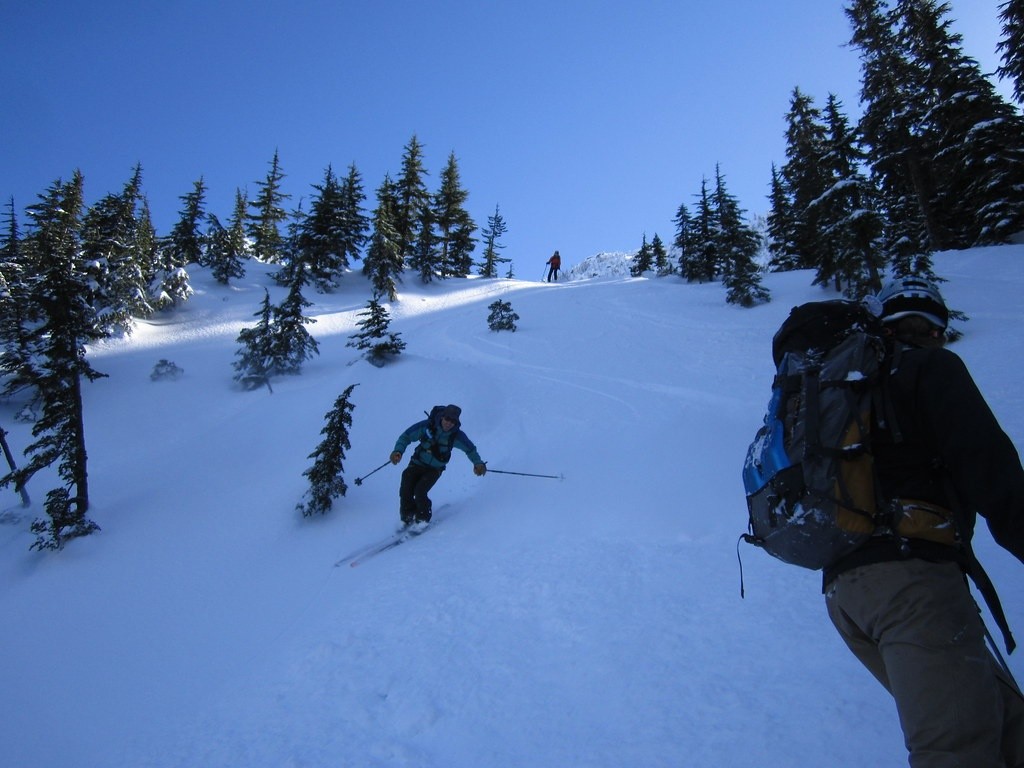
[875,276,948,329]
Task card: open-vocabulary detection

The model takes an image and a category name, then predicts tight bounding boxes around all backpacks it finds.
[743,299,970,570]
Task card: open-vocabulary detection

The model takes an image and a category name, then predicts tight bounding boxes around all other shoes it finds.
[408,520,428,535]
[399,520,414,532]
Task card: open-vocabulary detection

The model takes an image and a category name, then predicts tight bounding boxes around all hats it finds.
[442,404,461,422]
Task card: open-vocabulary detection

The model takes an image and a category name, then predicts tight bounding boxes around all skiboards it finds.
[336,504,456,567]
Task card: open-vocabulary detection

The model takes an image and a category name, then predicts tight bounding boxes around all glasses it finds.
[445,416,458,424]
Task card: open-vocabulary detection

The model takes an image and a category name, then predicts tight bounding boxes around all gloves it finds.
[473,464,486,476]
[390,451,401,465]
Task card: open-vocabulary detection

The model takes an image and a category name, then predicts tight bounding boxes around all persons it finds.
[390,404,486,535]
[822,278,1024,768]
[546,250,561,282]
